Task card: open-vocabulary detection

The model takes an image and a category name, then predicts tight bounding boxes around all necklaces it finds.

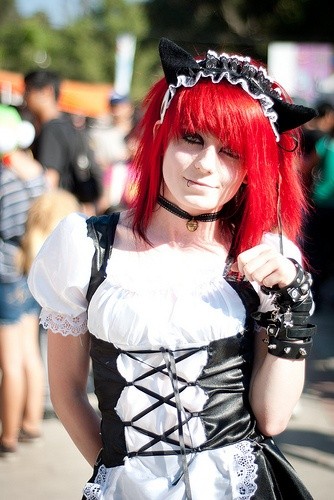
[155,194,232,232]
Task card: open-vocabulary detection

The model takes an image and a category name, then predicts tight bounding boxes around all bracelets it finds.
[253,257,316,359]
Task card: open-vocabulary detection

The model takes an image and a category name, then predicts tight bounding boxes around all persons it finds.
[25,54,317,500]
[95,89,333,201]
[0,109,47,455]
[26,66,106,420]
